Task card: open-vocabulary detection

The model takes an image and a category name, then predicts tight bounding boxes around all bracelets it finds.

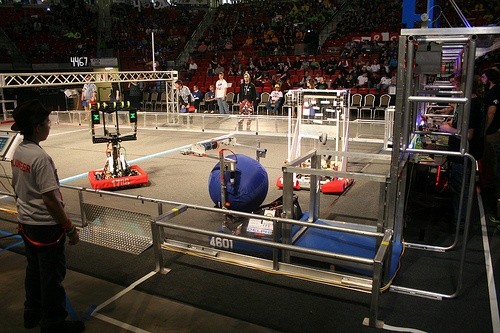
[64,219,79,240]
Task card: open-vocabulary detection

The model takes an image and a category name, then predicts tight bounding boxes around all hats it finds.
[12,99,53,131]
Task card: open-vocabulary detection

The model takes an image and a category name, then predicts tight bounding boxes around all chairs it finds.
[117,0,399,119]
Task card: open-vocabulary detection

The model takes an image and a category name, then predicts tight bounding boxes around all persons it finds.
[10,95,80,333]
[0,0,500,224]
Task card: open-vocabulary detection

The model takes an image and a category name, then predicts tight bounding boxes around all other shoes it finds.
[497,225,500,231]
[488,216,500,223]
[23,316,39,328]
[39,320,85,333]
